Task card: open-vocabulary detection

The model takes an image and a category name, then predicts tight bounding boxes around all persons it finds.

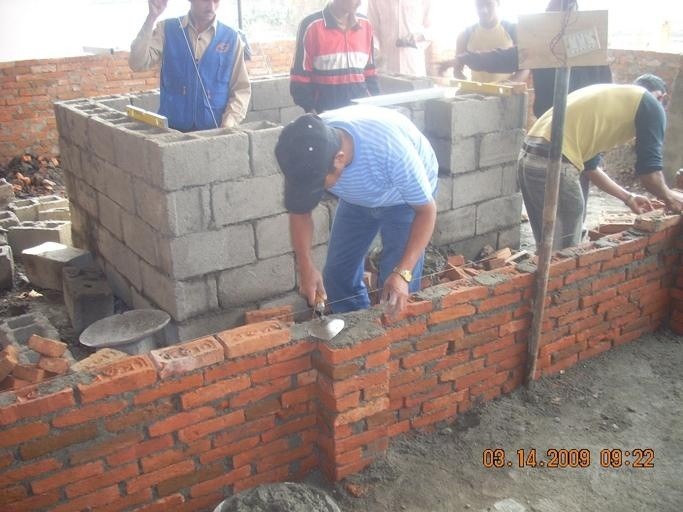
[127,0,253,132]
[427,1,616,221]
[365,0,445,83]
[513,72,682,254]
[287,0,384,116]
[451,1,531,88]
[273,101,439,315]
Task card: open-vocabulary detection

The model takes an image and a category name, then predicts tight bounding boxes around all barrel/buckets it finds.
[213,482,341,511]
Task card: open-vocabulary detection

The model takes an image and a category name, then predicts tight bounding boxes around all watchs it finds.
[393,267,412,283]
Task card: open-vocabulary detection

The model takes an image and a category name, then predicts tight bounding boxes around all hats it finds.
[274,111,338,215]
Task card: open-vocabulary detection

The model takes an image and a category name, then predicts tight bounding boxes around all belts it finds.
[521,141,571,163]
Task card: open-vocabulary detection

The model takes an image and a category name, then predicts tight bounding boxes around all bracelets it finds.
[624,191,635,207]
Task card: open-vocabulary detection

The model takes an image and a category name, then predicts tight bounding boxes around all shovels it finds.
[305,292,344,342]
